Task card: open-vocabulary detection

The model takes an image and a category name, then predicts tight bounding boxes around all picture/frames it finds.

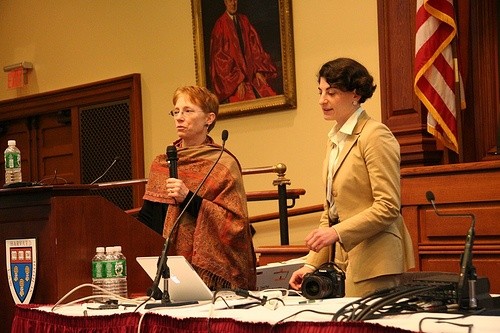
[190,0,297,120]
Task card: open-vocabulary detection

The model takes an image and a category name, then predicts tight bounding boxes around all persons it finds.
[137,86,258,290]
[289,58,417,298]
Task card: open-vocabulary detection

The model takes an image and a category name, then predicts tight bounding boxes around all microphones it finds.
[166,145,179,178]
[426,191,490,311]
[145,129,228,309]
[90,156,121,185]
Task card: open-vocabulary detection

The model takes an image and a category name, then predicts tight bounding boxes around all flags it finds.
[414,0,459,154]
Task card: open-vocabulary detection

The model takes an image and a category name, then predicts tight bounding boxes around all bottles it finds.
[101,247,120,303]
[114,248,129,298]
[92,246,107,303]
[4,140,23,185]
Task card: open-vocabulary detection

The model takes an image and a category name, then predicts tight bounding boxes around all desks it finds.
[11,291,500,333]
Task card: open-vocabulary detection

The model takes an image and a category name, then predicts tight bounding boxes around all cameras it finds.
[301,266,345,300]
